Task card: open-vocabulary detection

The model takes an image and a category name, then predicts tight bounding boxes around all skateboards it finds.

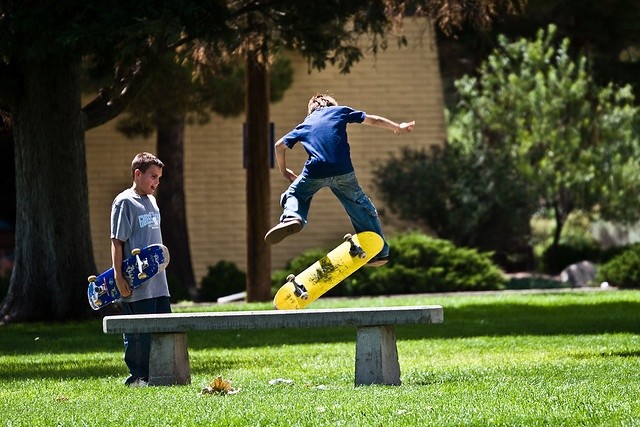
[274,231,385,310]
[88,242,170,310]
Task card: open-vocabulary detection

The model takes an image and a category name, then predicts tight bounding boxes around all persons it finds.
[110,152,171,387]
[263,93,415,267]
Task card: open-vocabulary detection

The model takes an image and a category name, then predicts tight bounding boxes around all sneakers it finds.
[264,218,303,245]
[365,257,389,267]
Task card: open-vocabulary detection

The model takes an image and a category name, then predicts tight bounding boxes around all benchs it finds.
[102,304,444,386]
